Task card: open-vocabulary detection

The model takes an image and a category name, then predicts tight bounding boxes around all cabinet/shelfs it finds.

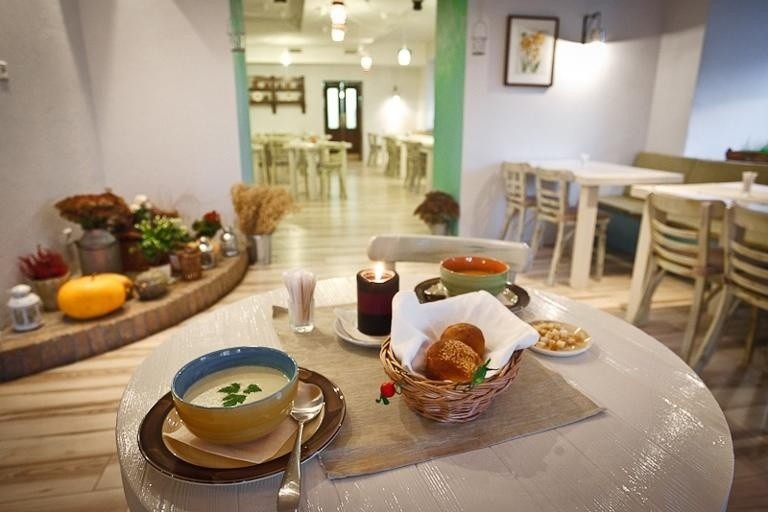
[247,75,309,115]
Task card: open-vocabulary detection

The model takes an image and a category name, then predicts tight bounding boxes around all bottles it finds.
[125,223,240,300]
[8,285,44,332]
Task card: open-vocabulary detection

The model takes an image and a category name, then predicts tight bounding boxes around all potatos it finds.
[56,272,132,320]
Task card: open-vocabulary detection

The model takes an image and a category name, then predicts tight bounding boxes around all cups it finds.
[741,181,752,194]
[288,297,316,336]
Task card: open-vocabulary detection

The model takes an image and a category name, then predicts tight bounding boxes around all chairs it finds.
[500,161,608,287]
[624,189,768,379]
[248,126,434,203]
[367,232,537,289]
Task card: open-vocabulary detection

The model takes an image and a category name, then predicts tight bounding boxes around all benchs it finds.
[597,154,768,292]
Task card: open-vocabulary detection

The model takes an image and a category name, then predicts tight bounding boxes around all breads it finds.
[441,323,485,357]
[426,338,484,384]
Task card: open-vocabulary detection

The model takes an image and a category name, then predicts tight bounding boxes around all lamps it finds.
[275,1,415,72]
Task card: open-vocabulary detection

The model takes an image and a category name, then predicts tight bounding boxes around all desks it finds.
[527,155,685,287]
[626,180,767,326]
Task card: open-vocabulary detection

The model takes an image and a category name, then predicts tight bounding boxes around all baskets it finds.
[379,336,525,423]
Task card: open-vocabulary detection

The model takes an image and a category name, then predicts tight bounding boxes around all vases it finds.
[427,221,450,235]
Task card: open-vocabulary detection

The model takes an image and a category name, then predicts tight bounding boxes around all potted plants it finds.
[228,179,299,268]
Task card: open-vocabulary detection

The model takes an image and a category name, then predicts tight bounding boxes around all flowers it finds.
[411,189,461,223]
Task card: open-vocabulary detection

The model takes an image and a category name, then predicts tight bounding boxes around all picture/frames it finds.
[504,12,561,87]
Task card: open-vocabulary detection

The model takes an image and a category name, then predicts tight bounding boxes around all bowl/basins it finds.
[439,255,510,301]
[170,343,299,448]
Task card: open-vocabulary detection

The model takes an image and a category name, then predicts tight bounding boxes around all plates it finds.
[135,366,348,487]
[331,310,388,348]
[412,276,531,313]
[525,318,591,357]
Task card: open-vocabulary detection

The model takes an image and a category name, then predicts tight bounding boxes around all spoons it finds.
[276,383,325,511]
